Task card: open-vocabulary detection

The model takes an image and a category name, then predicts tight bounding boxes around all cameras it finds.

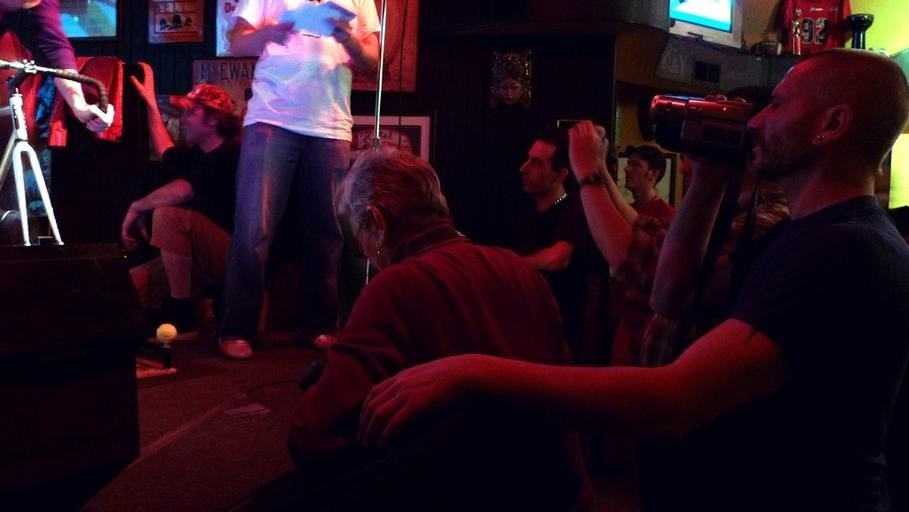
[649,94,755,152]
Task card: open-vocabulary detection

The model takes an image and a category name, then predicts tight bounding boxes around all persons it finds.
[1,1,908,512]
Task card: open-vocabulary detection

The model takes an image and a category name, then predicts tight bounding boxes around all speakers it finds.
[0,243,144,512]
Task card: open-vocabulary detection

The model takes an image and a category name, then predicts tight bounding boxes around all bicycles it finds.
[1,59,111,247]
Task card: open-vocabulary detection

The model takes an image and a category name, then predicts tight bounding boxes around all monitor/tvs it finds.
[667,0,745,49]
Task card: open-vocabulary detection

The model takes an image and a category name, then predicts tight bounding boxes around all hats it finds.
[170,84,234,114]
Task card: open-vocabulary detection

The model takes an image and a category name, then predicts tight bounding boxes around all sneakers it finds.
[306,332,338,348]
[219,336,253,358]
